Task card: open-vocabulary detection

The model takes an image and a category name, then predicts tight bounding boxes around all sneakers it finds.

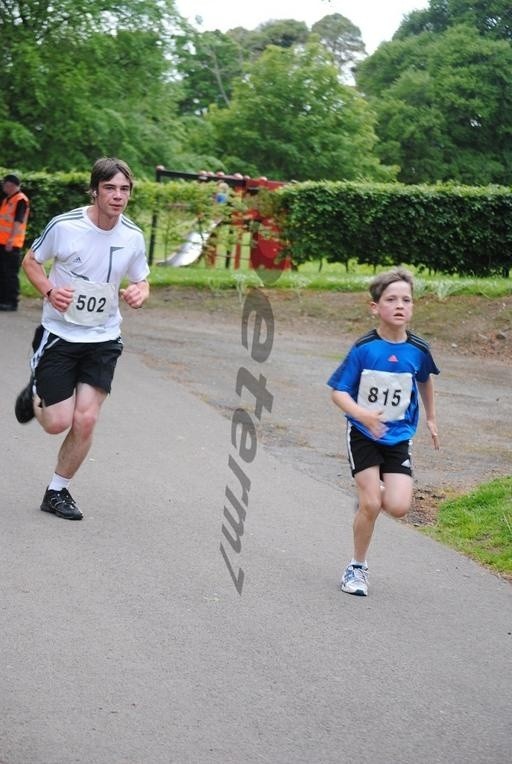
[342,563,368,595]
[15,381,34,423]
[40,485,83,520]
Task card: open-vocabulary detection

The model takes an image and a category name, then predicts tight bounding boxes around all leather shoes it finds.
[0,302,17,311]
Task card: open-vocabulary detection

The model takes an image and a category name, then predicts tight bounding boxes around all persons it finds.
[13,155,152,525]
[1,174,31,314]
[215,179,232,206]
[326,269,442,599]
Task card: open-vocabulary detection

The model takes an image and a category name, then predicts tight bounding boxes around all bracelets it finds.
[45,287,55,297]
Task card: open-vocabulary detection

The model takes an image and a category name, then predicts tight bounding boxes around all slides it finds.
[155,209,225,268]
